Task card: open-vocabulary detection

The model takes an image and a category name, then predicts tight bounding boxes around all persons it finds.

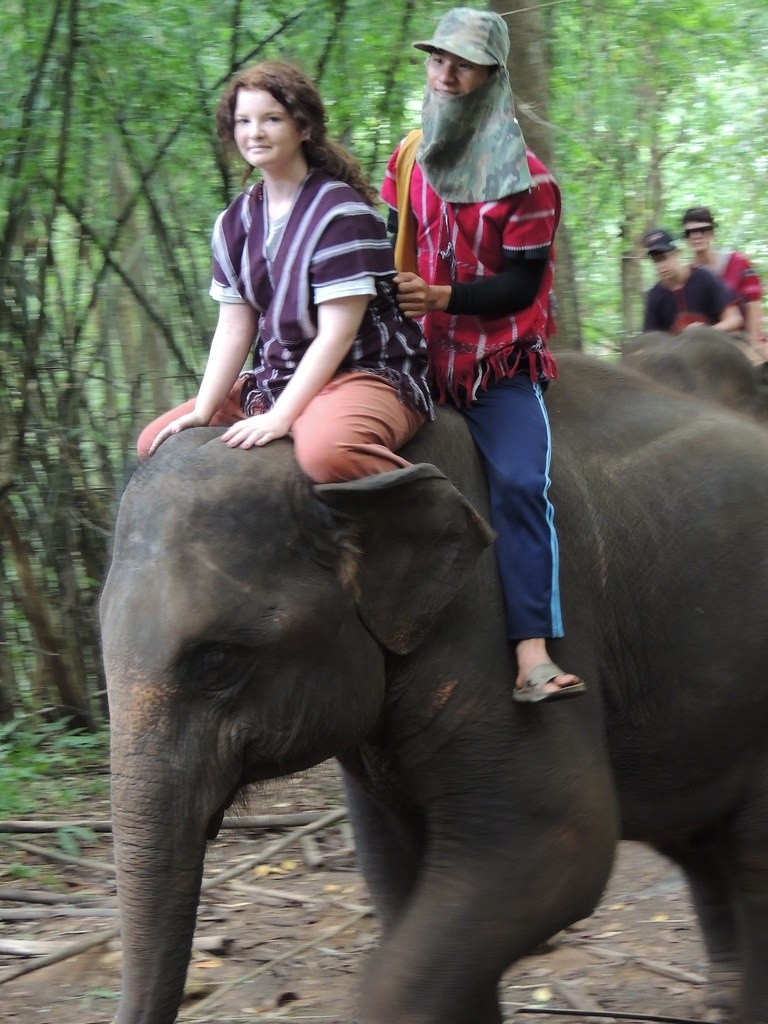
[378,7,588,704]
[640,208,767,343]
[132,64,439,485]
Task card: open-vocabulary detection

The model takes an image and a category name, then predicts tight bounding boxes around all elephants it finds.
[98,323,768,1023]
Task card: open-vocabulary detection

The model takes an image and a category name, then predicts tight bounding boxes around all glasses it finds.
[683,226,714,238]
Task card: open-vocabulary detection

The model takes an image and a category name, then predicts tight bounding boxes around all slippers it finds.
[512,661,587,702]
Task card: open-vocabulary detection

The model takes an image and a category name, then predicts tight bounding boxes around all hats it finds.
[642,229,676,255]
[413,7,535,205]
